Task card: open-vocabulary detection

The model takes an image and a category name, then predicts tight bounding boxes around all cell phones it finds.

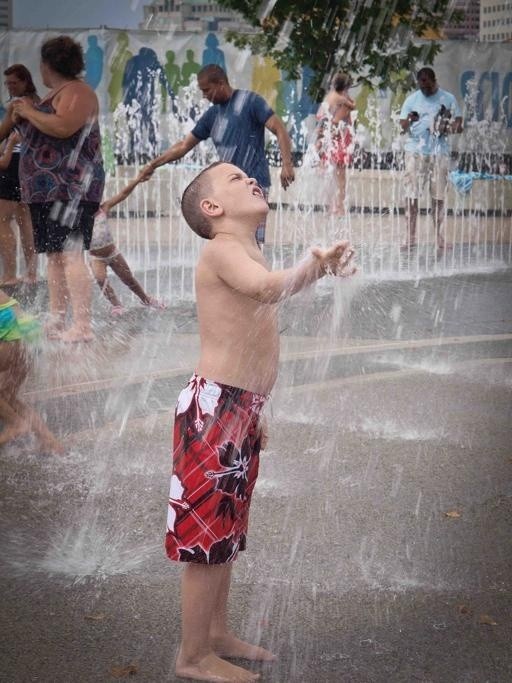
[411,112,419,121]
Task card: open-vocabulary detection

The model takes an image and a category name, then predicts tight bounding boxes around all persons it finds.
[250,52,413,153]
[1,62,45,288]
[460,67,512,132]
[14,35,105,345]
[85,170,169,314]
[315,69,358,214]
[82,28,227,162]
[0,290,71,458]
[138,62,296,253]
[398,68,463,251]
[166,158,360,682]
